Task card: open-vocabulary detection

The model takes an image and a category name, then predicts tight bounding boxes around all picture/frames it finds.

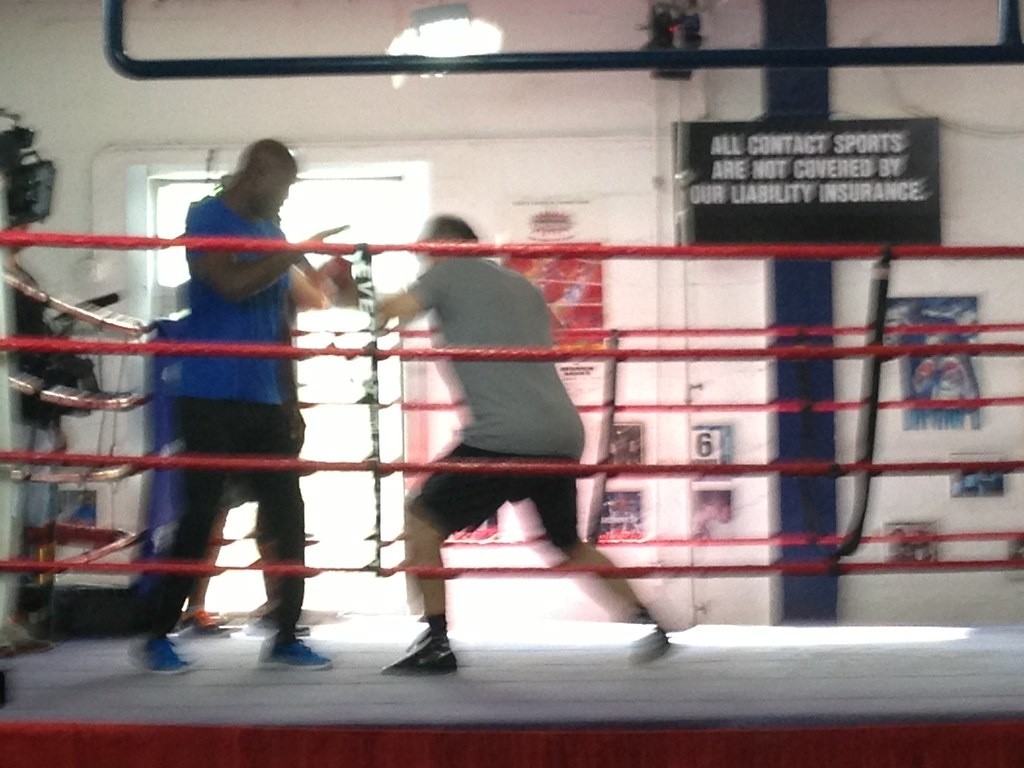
[612,421,645,465]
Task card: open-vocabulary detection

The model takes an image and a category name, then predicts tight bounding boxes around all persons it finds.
[375,216,670,674]
[132,140,381,670]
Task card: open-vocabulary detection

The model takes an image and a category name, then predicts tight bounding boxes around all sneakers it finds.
[127,634,190,676]
[259,634,334,671]
[382,635,457,675]
[624,609,668,667]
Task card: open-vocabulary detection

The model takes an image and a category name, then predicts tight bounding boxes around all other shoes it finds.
[193,610,219,633]
